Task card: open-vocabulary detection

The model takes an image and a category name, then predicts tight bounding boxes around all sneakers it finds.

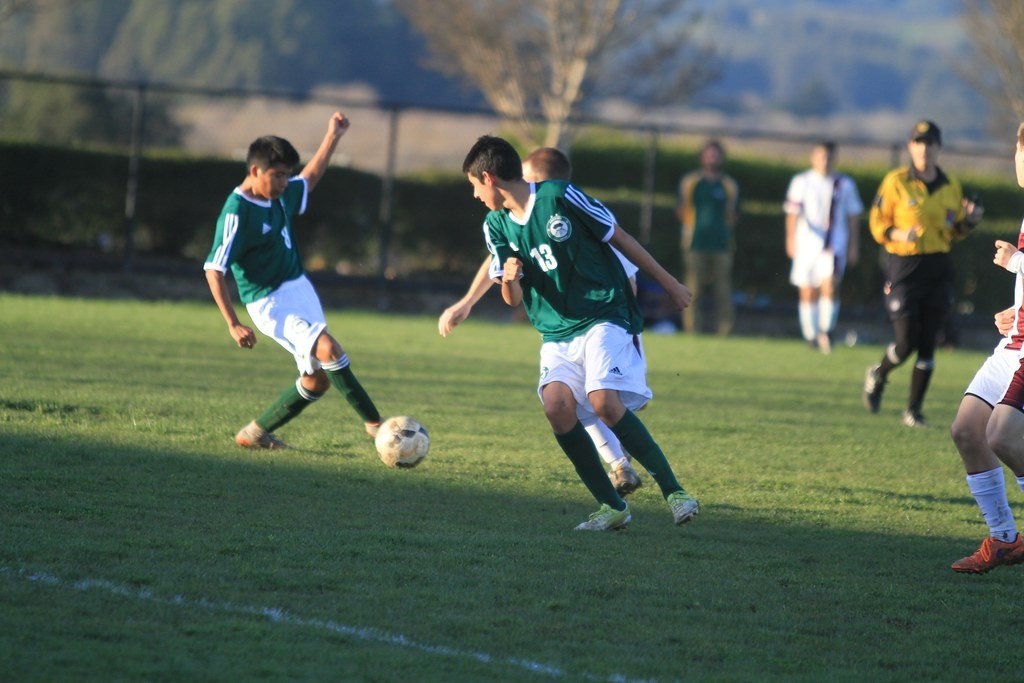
[236,421,293,450]
[608,467,643,496]
[666,489,700,524]
[903,410,926,428]
[864,363,888,412]
[573,499,633,532]
[364,417,385,437]
[951,532,1024,575]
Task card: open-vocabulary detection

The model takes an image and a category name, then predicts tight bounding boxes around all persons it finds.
[863,120,983,428]
[783,141,863,352]
[204,114,386,448]
[438,147,647,497]
[463,134,698,531]
[677,140,738,335]
[952,123,1024,572]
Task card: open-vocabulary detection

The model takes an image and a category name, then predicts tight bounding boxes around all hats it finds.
[908,121,939,142]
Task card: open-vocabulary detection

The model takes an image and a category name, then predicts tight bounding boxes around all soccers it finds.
[375,415,432,469]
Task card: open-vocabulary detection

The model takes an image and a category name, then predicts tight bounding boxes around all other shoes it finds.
[816,331,832,354]
